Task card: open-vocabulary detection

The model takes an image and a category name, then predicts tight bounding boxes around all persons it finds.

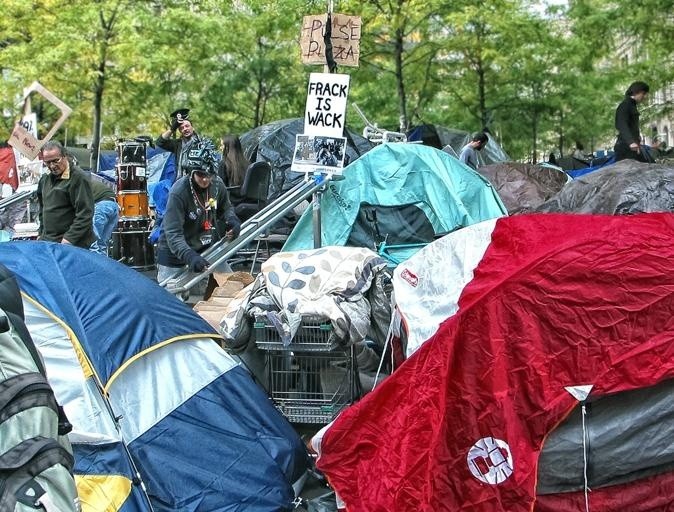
[37,140,95,249]
[156,107,199,180]
[88,179,120,256]
[156,149,240,303]
[460,132,489,171]
[614,82,649,162]
[218,135,258,207]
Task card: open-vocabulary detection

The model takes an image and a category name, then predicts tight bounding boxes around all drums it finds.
[115,142,146,164]
[117,192,148,219]
[118,219,149,231]
[112,232,154,267]
[116,164,147,192]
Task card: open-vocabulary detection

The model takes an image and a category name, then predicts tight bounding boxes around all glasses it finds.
[43,157,62,165]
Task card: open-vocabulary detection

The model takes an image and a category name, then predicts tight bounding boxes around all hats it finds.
[182,137,220,175]
[170,108,191,120]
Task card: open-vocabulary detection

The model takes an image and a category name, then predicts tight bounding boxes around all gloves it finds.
[184,250,209,274]
[225,216,241,240]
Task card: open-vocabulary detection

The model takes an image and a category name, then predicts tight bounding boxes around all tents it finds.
[280,144,509,267]
[307,212,674,512]
[0,240,313,512]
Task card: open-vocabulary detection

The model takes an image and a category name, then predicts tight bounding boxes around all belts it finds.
[96,196,118,202]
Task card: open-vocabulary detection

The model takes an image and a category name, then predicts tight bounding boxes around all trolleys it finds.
[252,302,356,429]
